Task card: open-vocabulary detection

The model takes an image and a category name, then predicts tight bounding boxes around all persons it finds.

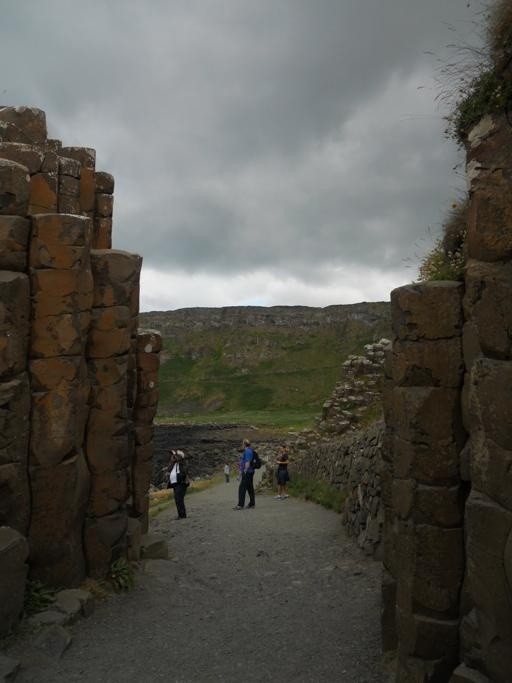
[232,463,241,481]
[167,448,191,520]
[272,446,290,500]
[223,463,230,484]
[231,438,256,510]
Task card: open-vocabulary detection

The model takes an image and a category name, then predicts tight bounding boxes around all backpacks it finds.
[250,451,261,468]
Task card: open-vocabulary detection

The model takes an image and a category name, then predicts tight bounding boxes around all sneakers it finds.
[245,504,255,508]
[232,504,244,510]
[272,495,289,500]
[175,514,186,520]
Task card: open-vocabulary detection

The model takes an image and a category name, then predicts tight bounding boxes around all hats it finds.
[171,449,184,459]
